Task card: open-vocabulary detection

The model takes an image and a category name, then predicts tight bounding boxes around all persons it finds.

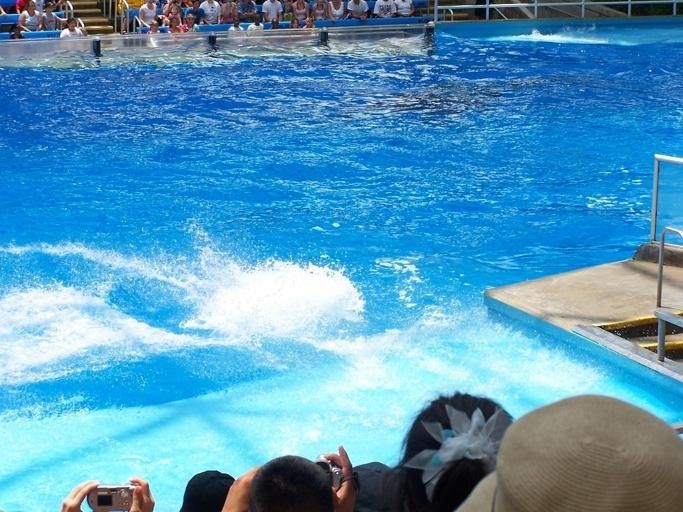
[121,1,416,47]
[0,1,89,50]
[180,446,355,512]
[63,478,152,512]
[350,394,683,512]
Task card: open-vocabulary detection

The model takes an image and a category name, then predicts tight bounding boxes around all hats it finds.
[454,394,682,512]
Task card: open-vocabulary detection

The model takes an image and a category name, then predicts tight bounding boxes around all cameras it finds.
[314,459,345,493]
[86,485,136,511]
